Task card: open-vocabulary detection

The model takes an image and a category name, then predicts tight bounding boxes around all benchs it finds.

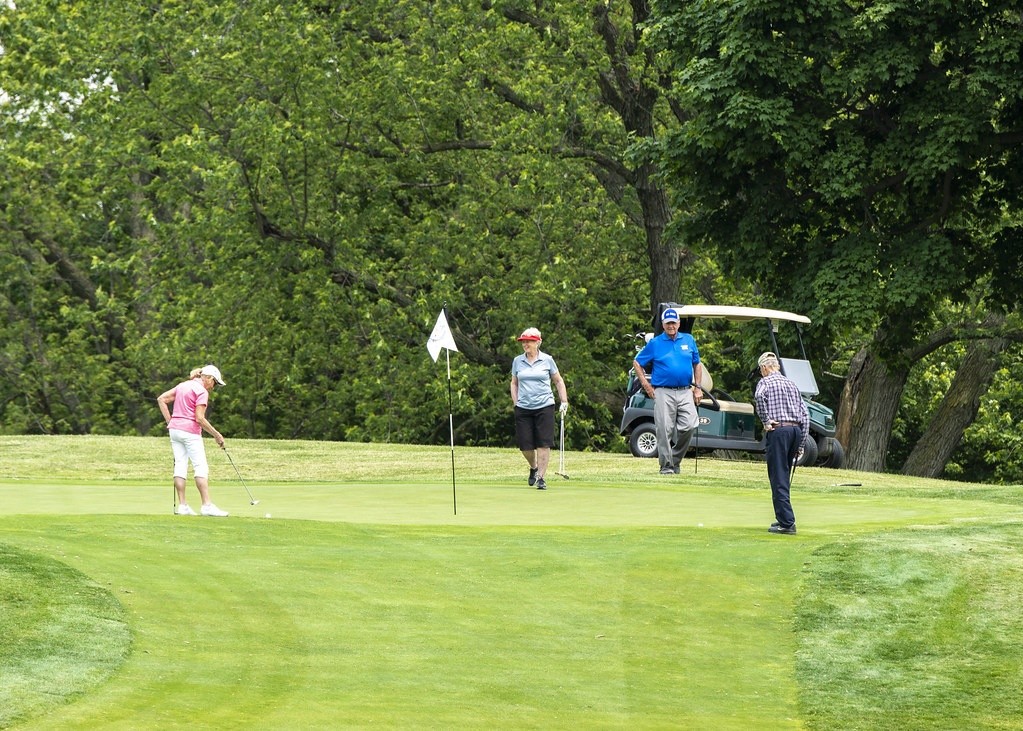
[689,383,754,415]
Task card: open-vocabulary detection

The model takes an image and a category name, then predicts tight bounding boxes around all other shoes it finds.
[659,466,680,474]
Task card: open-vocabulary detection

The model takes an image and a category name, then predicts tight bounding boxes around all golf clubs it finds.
[222,446,260,505]
[554,410,569,480]
[695,405,701,474]
[172,457,177,515]
[626,331,646,351]
[791,451,799,483]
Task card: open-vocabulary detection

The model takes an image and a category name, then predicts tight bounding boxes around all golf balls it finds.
[264,514,271,518]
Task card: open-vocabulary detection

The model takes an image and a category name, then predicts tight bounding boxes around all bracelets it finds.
[695,384,701,389]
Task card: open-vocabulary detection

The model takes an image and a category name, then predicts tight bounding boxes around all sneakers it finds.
[536,475,547,489]
[178,504,197,515]
[528,467,538,486]
[200,503,229,517]
[768,522,796,533]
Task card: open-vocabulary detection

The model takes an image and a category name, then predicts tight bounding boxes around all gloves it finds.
[559,402,568,416]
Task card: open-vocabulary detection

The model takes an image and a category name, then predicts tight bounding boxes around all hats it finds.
[517,335,541,341]
[758,352,777,366]
[201,365,226,386]
[662,308,679,323]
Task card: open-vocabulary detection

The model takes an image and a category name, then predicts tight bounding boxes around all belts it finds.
[664,385,690,390]
[773,422,799,427]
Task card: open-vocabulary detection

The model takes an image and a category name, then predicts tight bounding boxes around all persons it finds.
[633,309,703,475]
[755,352,810,533]
[510,327,568,490]
[157,365,228,516]
